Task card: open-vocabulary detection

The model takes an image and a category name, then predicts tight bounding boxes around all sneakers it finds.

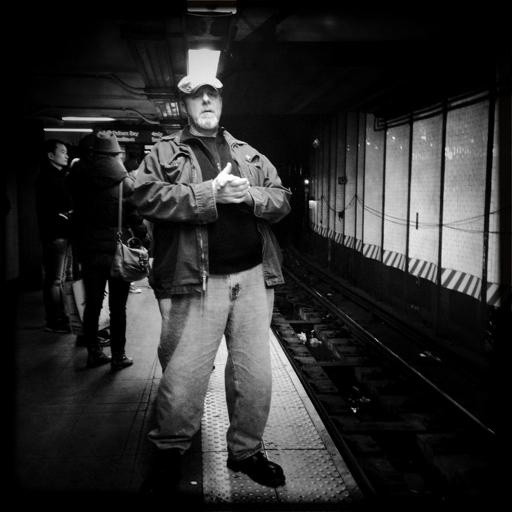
[129,286,142,293]
[46,324,71,333]
[75,334,112,347]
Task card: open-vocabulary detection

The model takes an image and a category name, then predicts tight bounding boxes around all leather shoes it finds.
[227,453,284,483]
[138,448,185,498]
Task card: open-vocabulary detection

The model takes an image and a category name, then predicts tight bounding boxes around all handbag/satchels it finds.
[72,279,111,331]
[111,241,150,282]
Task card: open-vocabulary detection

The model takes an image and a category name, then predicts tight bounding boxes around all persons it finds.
[72,129,137,372]
[123,48,292,492]
[35,140,151,348]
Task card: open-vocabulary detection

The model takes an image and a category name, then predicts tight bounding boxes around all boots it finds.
[85,342,111,368]
[110,339,133,368]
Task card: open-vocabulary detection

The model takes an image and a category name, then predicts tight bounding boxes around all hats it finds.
[90,131,126,153]
[177,73,223,97]
